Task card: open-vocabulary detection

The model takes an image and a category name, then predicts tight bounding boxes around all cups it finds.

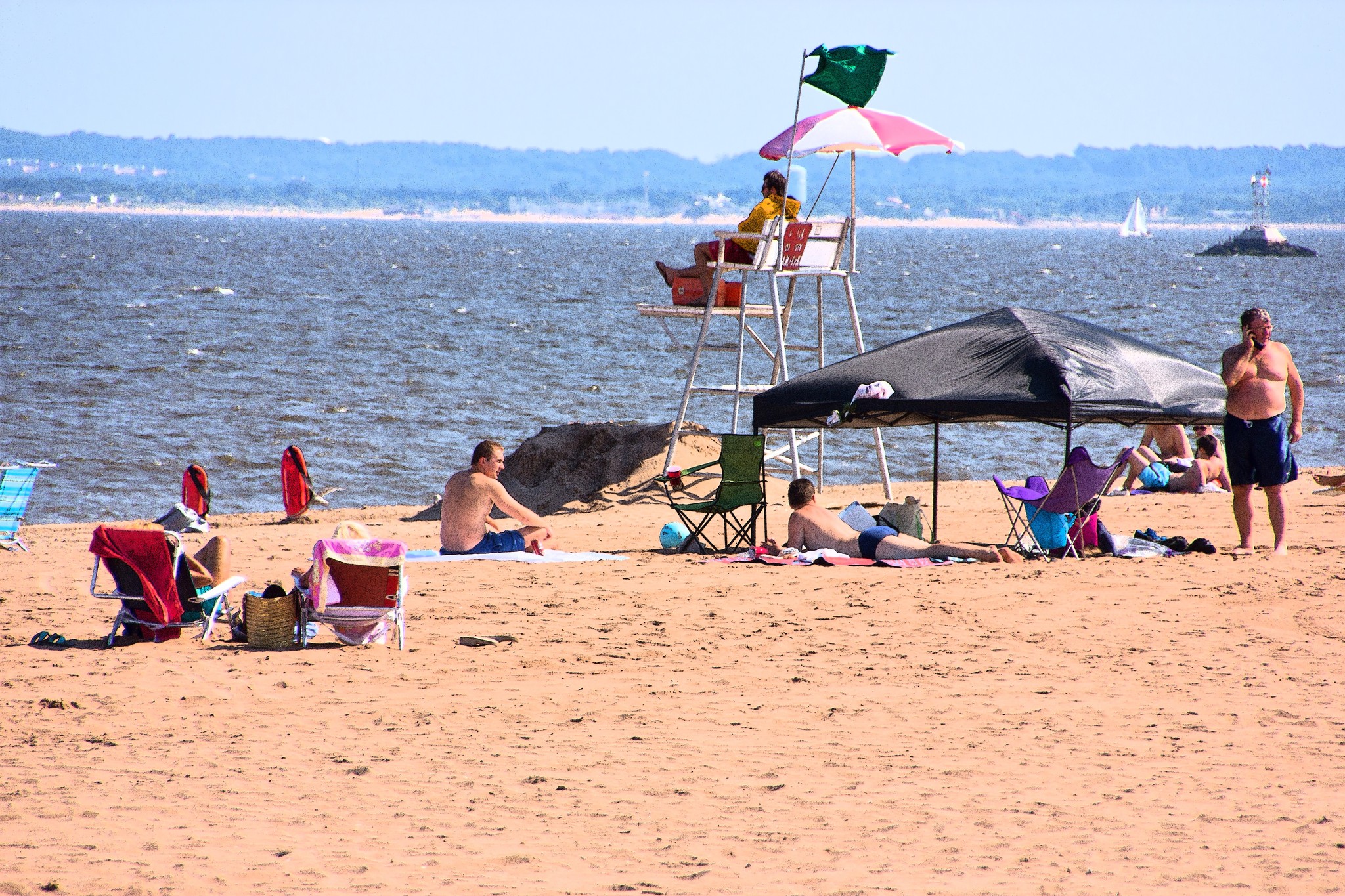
[749,546,769,558]
[666,466,680,486]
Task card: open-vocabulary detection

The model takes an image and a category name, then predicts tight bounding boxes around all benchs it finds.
[707,215,851,277]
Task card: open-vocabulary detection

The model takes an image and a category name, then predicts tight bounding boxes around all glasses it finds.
[761,186,769,191]
[1193,426,1210,431]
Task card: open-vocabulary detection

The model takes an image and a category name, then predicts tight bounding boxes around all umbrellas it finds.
[759,101,963,272]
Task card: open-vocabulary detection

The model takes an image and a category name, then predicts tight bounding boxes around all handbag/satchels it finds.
[1066,504,1098,551]
[873,514,900,533]
[838,502,877,531]
[1025,504,1076,550]
[878,502,935,544]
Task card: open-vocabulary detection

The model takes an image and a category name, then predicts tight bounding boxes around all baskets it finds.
[242,594,295,651]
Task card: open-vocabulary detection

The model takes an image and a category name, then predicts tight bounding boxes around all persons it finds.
[440,441,552,555]
[1098,434,1232,496]
[124,520,231,623]
[262,521,367,616]
[1164,424,1227,487]
[1312,473,1345,487]
[656,170,802,306]
[1221,308,1304,556]
[1139,424,1194,467]
[761,478,1022,563]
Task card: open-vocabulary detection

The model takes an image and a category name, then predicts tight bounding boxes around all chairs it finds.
[992,447,1135,563]
[0,459,58,552]
[89,526,246,647]
[292,538,407,650]
[655,434,768,556]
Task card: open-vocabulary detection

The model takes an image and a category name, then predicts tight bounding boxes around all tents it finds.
[752,305,1230,543]
